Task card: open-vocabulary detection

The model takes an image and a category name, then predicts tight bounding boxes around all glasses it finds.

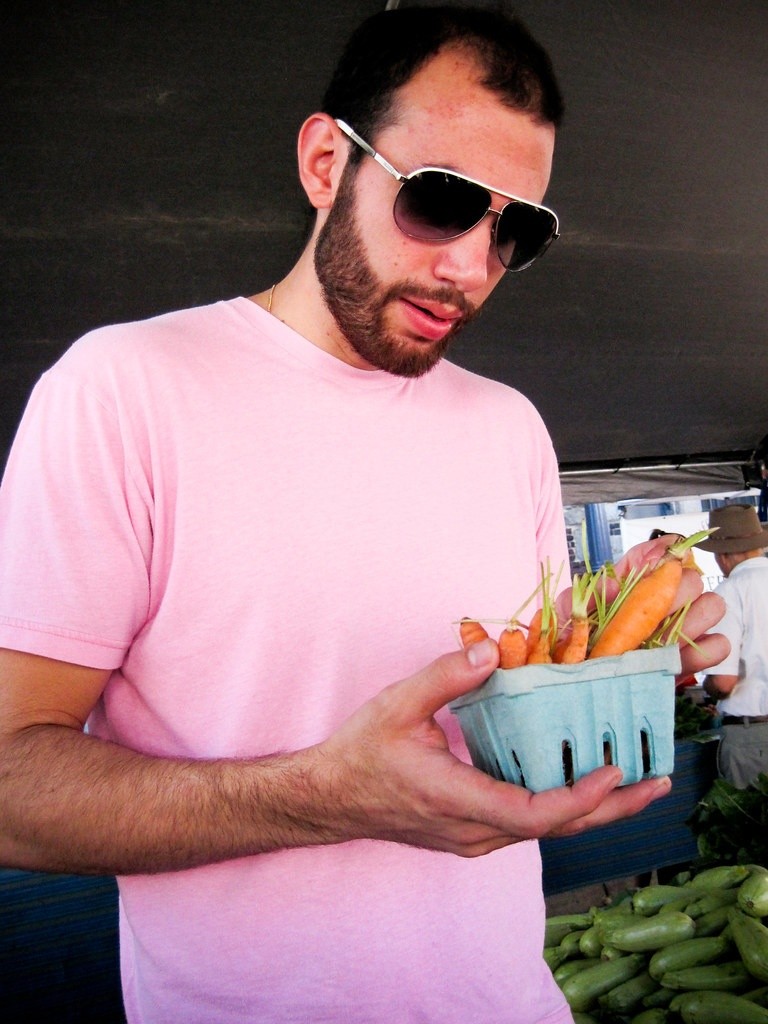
[333,119,562,274]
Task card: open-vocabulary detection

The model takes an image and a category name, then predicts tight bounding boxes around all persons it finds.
[691,503,768,790]
[649,529,698,686]
[0,0,731,1024]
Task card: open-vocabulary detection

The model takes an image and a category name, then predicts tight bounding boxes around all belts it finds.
[721,715,768,725]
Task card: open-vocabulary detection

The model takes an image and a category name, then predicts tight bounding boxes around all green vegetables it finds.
[685,773,768,875]
[673,694,710,739]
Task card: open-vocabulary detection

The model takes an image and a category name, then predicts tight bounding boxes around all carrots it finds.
[459,558,683,672]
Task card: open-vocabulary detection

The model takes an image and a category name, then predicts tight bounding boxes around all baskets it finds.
[449,642,683,800]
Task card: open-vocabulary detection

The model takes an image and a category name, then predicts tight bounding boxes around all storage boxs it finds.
[443,644,682,794]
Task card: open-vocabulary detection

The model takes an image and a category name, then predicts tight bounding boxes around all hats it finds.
[692,503,768,553]
